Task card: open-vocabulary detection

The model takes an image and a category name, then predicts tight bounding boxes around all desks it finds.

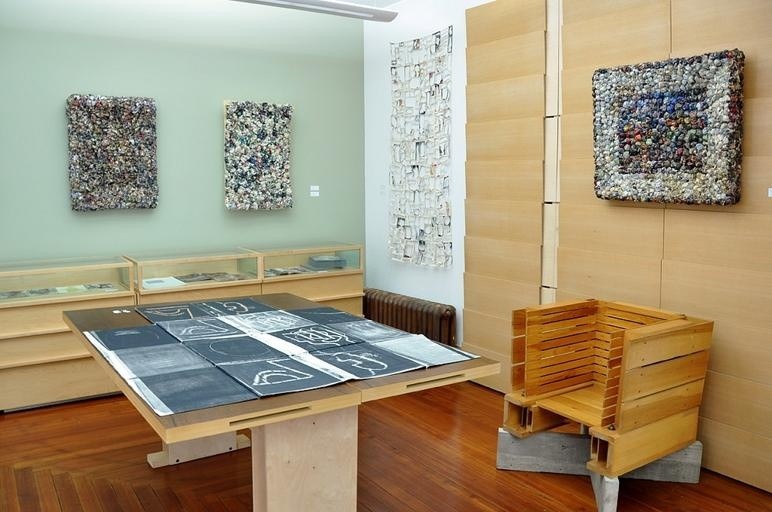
[56,292,503,512]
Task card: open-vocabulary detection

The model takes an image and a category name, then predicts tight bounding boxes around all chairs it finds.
[495,297,716,511]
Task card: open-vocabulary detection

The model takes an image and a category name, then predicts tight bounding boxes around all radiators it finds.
[361,286,456,343]
